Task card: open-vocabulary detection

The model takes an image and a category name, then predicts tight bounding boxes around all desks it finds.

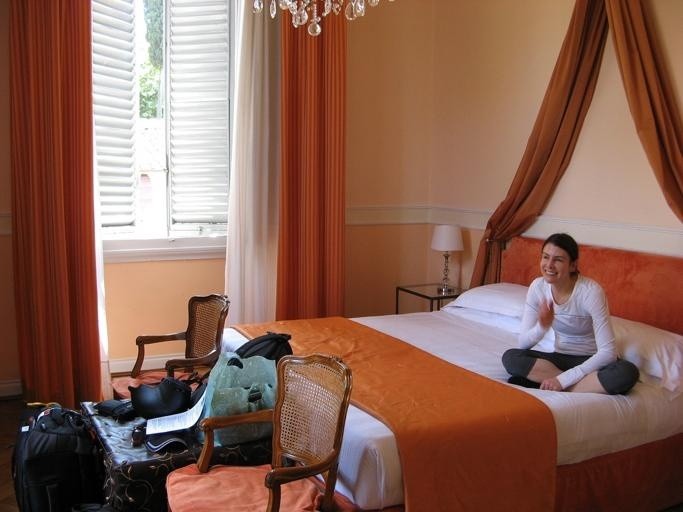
[79,399,196,512]
[394,283,465,313]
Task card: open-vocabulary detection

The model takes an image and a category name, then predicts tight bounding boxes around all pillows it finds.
[436,279,681,398]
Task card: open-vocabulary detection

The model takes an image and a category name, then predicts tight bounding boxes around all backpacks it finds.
[190,331,293,414]
[11,406,106,512]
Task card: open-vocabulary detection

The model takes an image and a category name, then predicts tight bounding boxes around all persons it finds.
[500,232,640,394]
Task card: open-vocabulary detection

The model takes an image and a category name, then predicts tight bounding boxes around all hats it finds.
[146,432,190,452]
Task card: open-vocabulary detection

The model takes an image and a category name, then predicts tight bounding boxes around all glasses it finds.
[131,420,145,446]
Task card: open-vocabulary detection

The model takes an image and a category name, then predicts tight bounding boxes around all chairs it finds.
[167,353,353,510]
[115,293,229,398]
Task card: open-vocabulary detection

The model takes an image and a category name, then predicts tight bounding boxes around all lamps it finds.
[430,223,465,294]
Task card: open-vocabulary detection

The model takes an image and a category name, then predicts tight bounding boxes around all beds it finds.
[222,234,682,512]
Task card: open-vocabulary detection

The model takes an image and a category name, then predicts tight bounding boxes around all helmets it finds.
[130,378,205,421]
